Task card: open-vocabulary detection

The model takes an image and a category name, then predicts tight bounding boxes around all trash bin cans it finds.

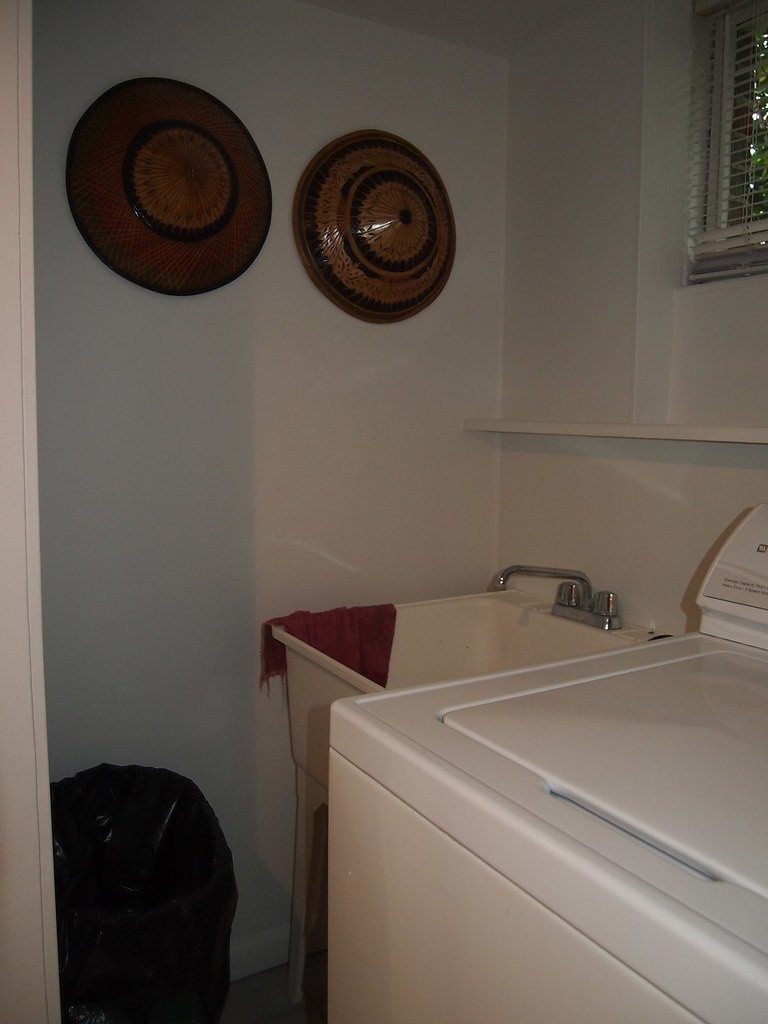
[52,762,237,1024]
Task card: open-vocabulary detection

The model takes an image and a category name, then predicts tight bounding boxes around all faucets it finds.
[491,563,594,612]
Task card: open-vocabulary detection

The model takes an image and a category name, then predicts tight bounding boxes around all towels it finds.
[258,601,397,698]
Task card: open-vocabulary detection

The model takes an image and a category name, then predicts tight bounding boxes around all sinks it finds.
[271,592,643,694]
